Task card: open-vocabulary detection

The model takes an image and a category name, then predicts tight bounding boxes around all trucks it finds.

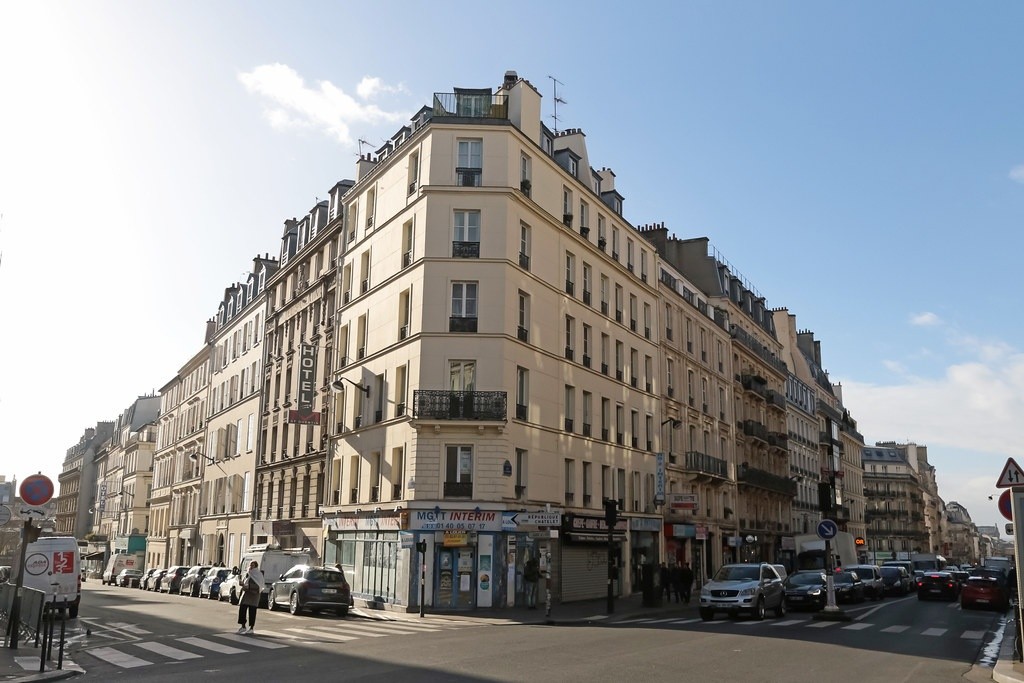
[910,553,946,568]
[984,557,1011,574]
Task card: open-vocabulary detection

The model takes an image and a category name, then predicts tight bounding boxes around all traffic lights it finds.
[605,500,620,526]
[817,481,834,512]
[415,542,427,553]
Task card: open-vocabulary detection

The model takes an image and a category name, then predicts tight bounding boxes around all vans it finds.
[102,553,138,586]
[21,535,81,619]
[230,543,311,608]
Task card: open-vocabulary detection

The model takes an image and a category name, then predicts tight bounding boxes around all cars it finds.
[962,573,1007,613]
[116,569,144,587]
[783,570,840,609]
[913,569,960,604]
[80,568,87,582]
[878,561,917,596]
[943,563,982,582]
[834,571,866,599]
[139,566,240,601]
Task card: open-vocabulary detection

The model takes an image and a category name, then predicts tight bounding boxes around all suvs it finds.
[267,563,351,619]
[851,565,883,594]
[698,561,787,620]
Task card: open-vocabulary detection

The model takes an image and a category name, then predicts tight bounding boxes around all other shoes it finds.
[237,627,254,633]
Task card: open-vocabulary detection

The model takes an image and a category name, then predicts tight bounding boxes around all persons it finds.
[660,561,694,603]
[524,557,546,608]
[235,561,265,635]
